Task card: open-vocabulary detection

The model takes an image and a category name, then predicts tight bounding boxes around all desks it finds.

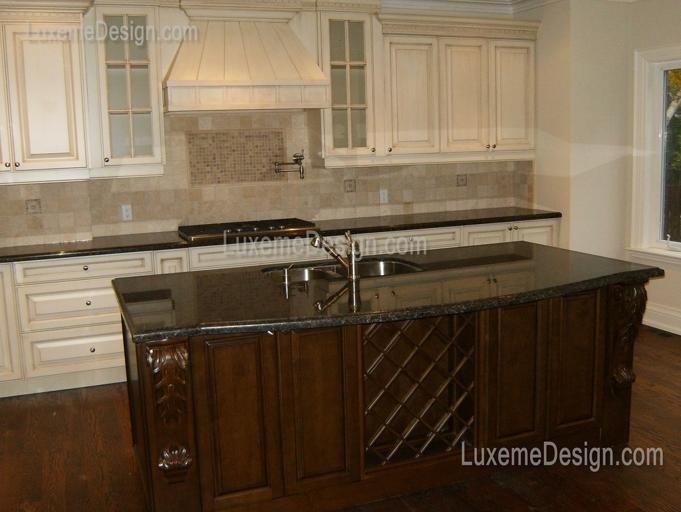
[110,240,665,512]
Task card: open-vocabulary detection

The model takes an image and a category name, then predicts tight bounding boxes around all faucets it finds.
[275,166,305,181]
[311,231,360,279]
[314,280,360,313]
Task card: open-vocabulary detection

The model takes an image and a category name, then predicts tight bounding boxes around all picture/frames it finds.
[630,43,681,251]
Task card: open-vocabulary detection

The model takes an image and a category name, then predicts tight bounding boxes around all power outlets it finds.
[122,205,132,221]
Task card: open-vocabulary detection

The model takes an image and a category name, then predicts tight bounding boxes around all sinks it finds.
[264,266,336,283]
[325,261,418,279]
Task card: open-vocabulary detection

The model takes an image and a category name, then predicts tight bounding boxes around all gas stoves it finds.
[175,217,322,241]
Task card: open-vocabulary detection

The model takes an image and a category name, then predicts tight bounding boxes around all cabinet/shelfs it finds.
[0,248,189,382]
[319,10,379,158]
[467,225,553,246]
[440,37,535,152]
[96,5,161,167]
[0,8,87,173]
[383,34,440,156]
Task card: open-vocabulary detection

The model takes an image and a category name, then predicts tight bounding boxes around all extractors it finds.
[162,17,330,116]
